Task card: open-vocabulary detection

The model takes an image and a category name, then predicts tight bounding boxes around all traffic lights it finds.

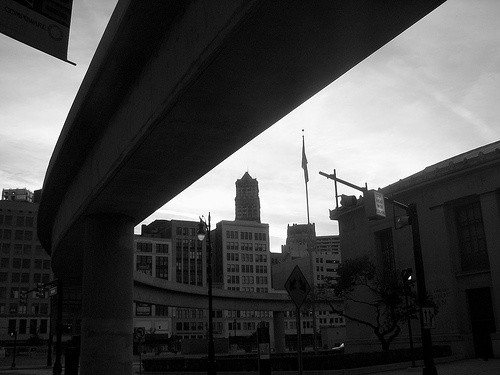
[9,330,15,337]
[19,290,27,307]
[36,282,45,298]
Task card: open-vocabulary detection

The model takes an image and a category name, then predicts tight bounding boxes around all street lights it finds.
[197,211,215,375]
[402,269,416,366]
